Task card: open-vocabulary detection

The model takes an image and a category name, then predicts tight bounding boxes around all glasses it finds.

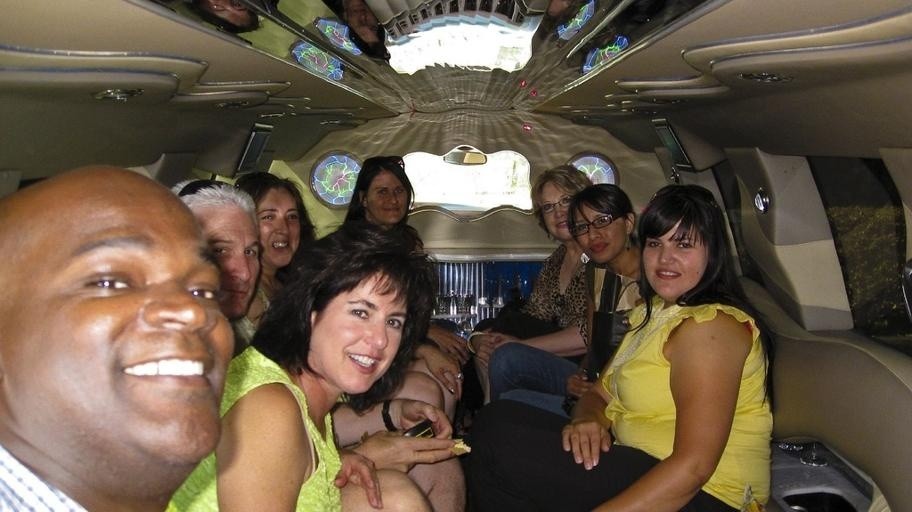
[645,182,721,212]
[358,156,405,174]
[569,210,628,236]
[177,179,239,196]
[539,195,576,214]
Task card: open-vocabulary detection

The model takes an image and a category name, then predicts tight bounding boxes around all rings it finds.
[453,372,462,379]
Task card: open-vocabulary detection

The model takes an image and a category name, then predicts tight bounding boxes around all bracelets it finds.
[382,399,398,431]
[467,331,483,353]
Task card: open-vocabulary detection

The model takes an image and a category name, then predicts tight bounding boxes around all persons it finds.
[235,171,463,432]
[345,155,471,363]
[465,183,773,511]
[171,176,470,510]
[0,163,233,512]
[164,222,434,512]
[461,164,594,414]
[488,183,657,421]
